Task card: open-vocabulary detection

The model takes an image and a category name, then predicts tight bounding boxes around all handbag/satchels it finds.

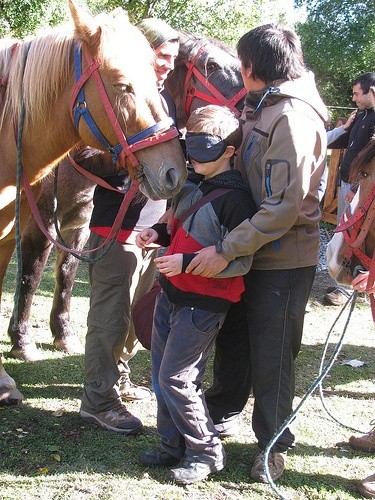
[133,280,163,350]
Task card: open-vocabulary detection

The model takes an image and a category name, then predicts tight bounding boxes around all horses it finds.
[325,129,375,296]
[0,0,247,408]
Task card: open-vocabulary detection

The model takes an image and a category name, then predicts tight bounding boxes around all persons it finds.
[349,271,375,497]
[135,105,255,485]
[71,19,181,435]
[159,25,331,482]
[320,72,375,306]
[327,110,357,145]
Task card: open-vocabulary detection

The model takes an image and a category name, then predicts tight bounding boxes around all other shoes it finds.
[357,472,375,498]
[349,418,375,452]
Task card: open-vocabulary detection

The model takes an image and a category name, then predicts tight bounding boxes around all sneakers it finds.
[120,375,152,402]
[215,415,242,436]
[324,290,349,306]
[138,448,180,467]
[170,451,227,484]
[250,447,288,483]
[80,395,143,434]
[327,286,365,303]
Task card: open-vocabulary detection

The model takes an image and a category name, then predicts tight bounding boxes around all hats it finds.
[133,16,180,51]
